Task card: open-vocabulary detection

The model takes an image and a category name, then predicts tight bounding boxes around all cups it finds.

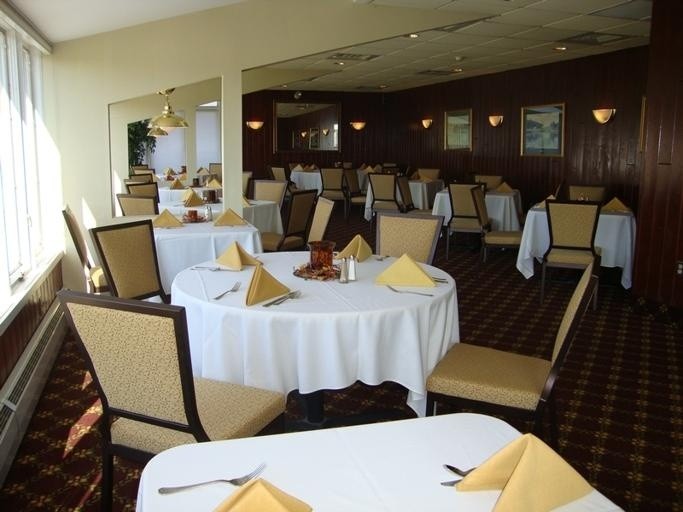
[207,190,215,201]
[187,210,198,220]
[192,178,198,186]
[306,240,337,269]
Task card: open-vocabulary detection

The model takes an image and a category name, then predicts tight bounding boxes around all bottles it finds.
[204,205,211,222]
[338,254,356,283]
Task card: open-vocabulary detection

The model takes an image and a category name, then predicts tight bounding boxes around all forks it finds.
[188,264,222,272]
[429,276,450,285]
[157,462,267,496]
[213,280,241,301]
[374,252,389,263]
[385,284,434,298]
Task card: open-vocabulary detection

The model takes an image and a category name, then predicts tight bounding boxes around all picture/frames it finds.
[309,127,320,151]
[443,108,473,152]
[520,102,565,158]
[292,128,301,150]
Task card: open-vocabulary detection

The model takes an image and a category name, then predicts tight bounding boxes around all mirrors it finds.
[273,98,342,153]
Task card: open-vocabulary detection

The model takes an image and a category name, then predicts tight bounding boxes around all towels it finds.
[601,197,633,213]
[216,476,314,512]
[455,433,593,512]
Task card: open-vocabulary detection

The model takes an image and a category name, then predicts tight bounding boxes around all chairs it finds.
[55,290,286,512]
[87,218,168,304]
[425,258,602,452]
[116,163,159,216]
[64,203,112,294]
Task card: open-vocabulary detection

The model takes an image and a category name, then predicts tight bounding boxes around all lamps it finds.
[351,122,366,130]
[422,119,433,129]
[147,88,190,128]
[322,129,328,135]
[247,121,264,129]
[592,109,613,124]
[489,116,503,127]
[301,132,307,137]
[146,128,167,136]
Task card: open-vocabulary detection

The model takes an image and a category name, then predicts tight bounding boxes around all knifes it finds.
[261,288,303,309]
